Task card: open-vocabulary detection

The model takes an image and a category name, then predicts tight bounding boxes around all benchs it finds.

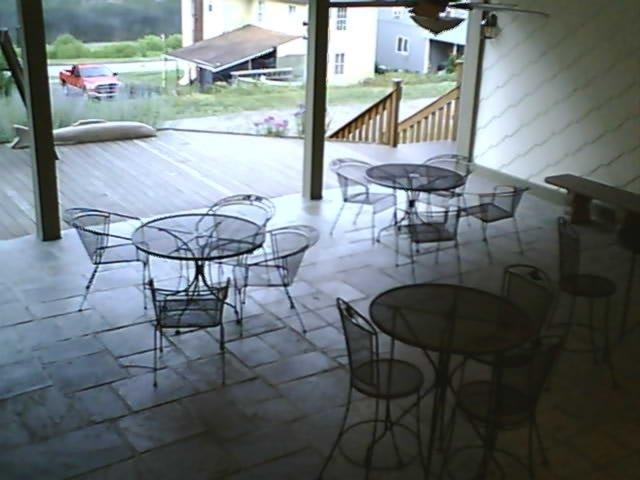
[545,173,640,238]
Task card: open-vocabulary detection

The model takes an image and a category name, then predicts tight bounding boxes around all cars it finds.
[178,59,268,86]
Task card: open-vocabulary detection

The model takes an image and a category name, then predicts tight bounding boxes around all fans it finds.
[328,0,546,17]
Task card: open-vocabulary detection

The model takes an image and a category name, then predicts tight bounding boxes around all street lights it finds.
[159,31,169,87]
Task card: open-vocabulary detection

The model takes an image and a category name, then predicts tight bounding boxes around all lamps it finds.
[411,15,464,38]
[480,14,501,39]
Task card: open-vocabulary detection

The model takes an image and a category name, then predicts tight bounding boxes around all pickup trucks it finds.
[59,63,123,102]
